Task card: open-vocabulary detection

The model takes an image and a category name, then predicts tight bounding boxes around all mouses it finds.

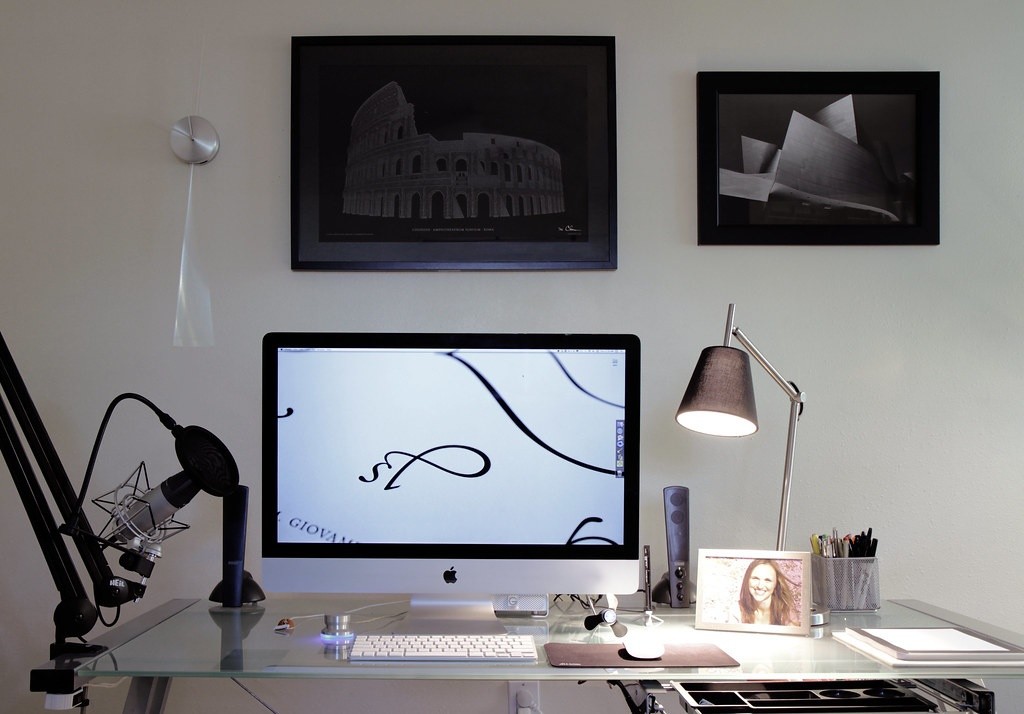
[621,630,665,659]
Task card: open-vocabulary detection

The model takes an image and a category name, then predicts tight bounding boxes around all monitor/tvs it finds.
[260,332,642,634]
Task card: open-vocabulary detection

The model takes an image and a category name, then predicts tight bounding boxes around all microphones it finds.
[111,470,200,544]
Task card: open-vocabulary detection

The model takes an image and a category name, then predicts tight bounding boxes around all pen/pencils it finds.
[810,526,880,613]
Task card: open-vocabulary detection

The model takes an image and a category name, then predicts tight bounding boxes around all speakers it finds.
[207,485,265,608]
[651,486,696,608]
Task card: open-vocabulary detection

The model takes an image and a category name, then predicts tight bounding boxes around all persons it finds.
[721,559,801,627]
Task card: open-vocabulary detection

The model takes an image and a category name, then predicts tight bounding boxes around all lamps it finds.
[675,304,830,627]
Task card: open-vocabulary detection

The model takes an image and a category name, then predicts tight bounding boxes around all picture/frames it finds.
[695,549,812,636]
[697,72,939,245]
[290,37,619,269]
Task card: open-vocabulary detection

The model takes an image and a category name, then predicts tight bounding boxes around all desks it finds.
[31,597,1024,714]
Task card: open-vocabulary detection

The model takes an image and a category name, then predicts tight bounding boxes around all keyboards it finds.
[346,635,538,662]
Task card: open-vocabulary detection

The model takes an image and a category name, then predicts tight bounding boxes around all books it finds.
[832,626,1024,668]
